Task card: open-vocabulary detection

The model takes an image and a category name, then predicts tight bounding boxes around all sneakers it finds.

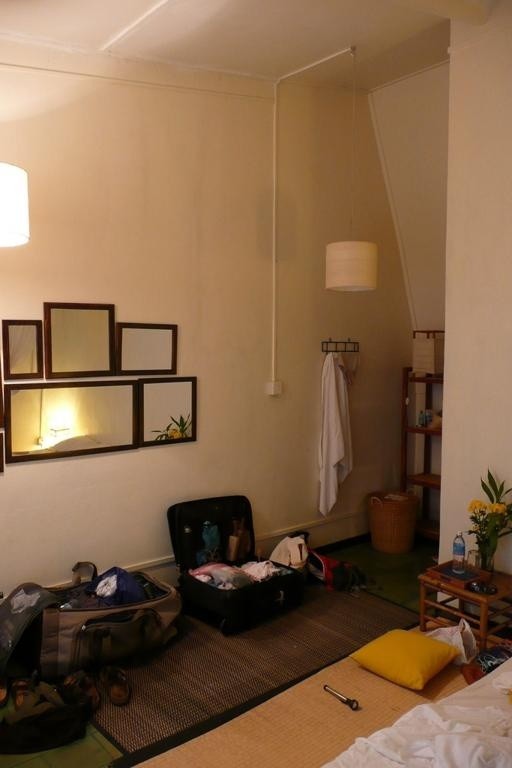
[81,674,101,709]
[99,670,130,705]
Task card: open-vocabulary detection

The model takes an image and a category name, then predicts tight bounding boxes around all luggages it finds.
[167,494,304,638]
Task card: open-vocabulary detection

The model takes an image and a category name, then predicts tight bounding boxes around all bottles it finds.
[418,410,425,427]
[452,530,466,575]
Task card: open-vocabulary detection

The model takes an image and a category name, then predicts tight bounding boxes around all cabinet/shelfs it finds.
[398,366,444,541]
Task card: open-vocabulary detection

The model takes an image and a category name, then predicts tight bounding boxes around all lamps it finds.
[49,428,69,437]
[0,161,32,247]
[325,241,378,291]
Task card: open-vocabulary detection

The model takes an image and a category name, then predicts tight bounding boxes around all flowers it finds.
[466,466,512,560]
[150,412,193,440]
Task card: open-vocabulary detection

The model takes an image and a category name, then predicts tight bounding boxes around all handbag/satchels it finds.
[0,569,181,672]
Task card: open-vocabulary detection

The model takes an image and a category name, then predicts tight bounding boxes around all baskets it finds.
[366,487,418,555]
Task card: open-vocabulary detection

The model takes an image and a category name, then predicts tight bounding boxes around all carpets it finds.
[91,585,420,756]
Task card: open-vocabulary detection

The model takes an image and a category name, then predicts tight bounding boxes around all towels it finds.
[317,352,344,520]
[335,353,353,488]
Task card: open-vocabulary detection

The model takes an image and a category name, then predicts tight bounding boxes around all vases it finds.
[476,557,494,572]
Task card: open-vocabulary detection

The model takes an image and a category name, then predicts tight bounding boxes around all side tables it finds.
[417,559,511,652]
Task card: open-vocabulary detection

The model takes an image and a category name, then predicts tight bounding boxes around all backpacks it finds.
[0,663,100,756]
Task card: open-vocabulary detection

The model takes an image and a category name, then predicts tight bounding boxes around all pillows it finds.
[348,628,463,690]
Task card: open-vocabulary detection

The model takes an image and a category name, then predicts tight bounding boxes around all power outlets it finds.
[268,382,283,396]
[37,437,44,445]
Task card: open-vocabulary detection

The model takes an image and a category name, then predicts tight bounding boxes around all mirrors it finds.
[3,319,43,381]
[139,376,197,448]
[118,321,178,376]
[4,381,138,463]
[43,302,116,379]
